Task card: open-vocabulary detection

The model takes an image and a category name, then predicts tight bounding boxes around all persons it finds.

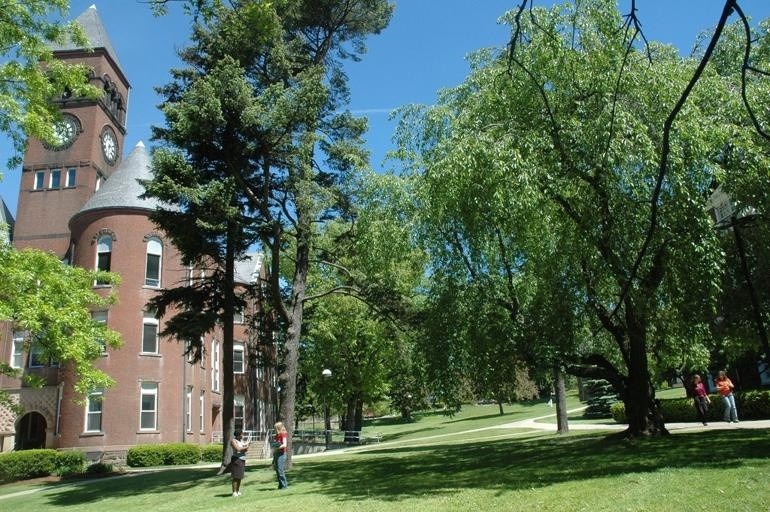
[687,374,712,427]
[268,421,289,491]
[230,429,252,499]
[714,370,740,423]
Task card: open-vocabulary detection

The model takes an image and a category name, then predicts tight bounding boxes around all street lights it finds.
[321,369,332,451]
